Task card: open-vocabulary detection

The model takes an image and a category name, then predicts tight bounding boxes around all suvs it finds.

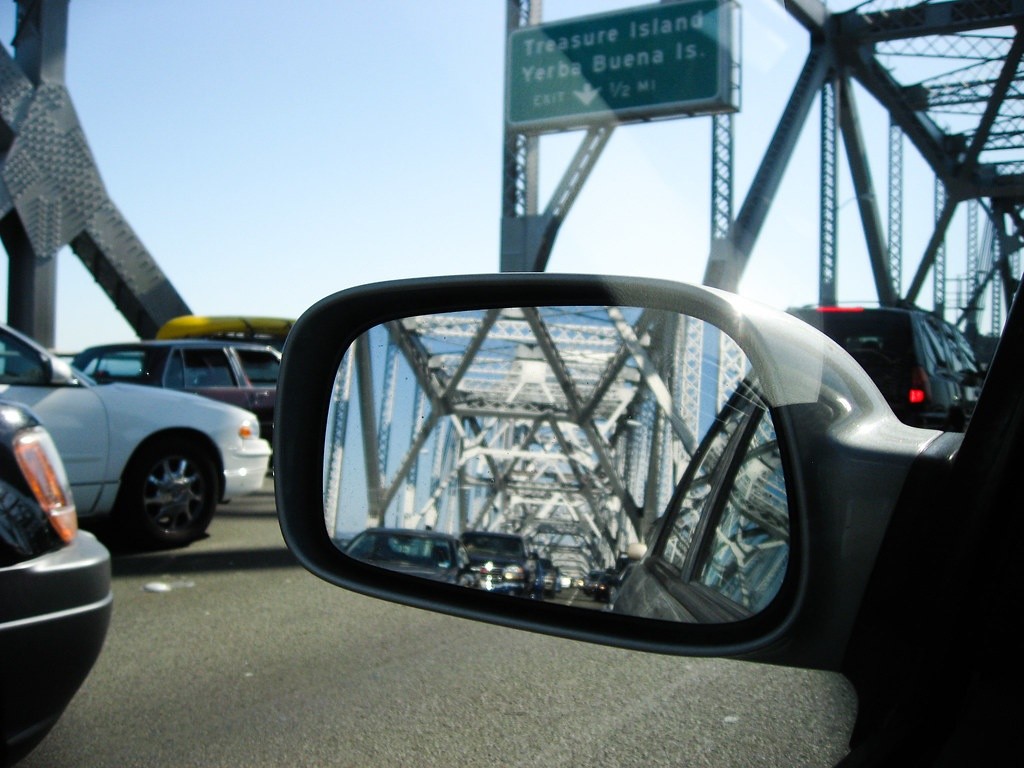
[72,335,280,485]
[457,528,544,606]
[778,298,985,436]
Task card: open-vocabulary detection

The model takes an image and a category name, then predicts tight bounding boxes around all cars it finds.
[523,552,622,602]
[0,395,115,768]
[0,321,275,553]
[340,529,479,597]
[274,268,1024,768]
